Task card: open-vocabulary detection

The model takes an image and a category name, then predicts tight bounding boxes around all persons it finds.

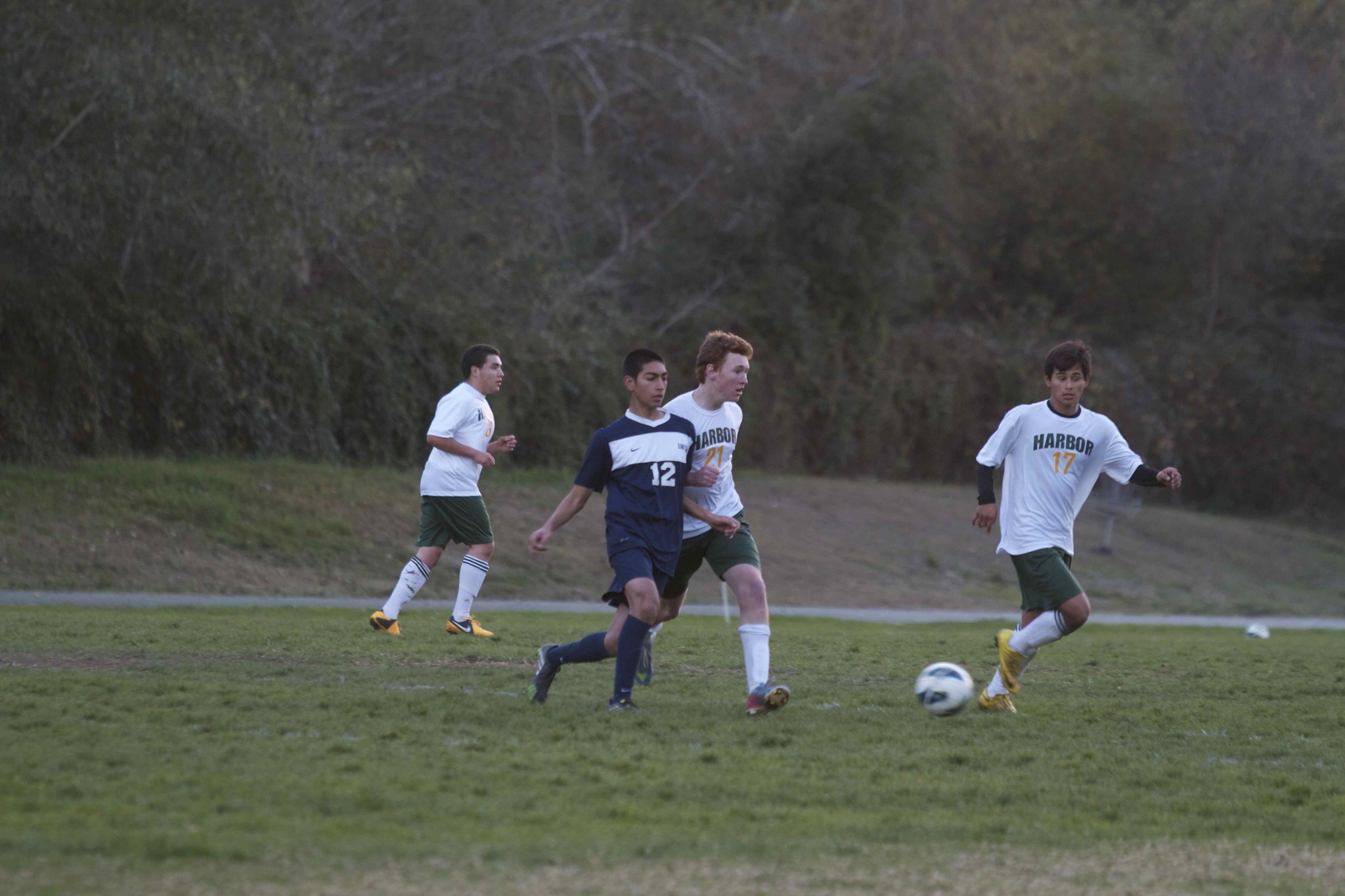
[968,338,1183,715]
[525,346,742,714]
[367,341,517,641]
[634,329,794,718]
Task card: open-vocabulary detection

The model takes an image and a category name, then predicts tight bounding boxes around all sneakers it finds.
[978,689,1017,715]
[446,615,494,638]
[526,643,561,707]
[746,682,791,717]
[994,628,1028,695]
[369,607,403,638]
[635,631,656,685]
[607,699,647,716]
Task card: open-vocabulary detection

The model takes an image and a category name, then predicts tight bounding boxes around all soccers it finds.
[915,661,976,715]
